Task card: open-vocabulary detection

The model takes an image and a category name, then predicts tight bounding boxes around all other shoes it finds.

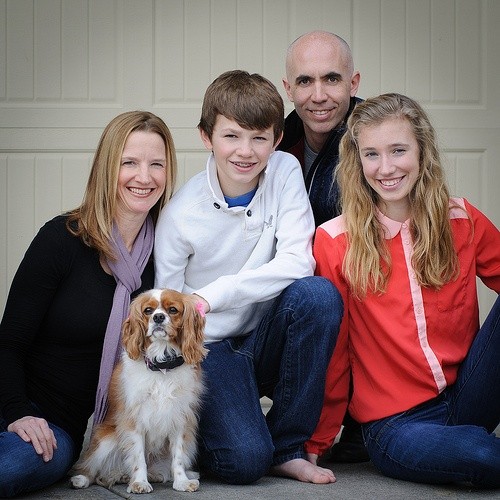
[332,428,369,464]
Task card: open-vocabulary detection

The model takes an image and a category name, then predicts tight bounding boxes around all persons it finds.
[152,69,343,485]
[272,30,377,232]
[314,93,500,493]
[0,111,178,499]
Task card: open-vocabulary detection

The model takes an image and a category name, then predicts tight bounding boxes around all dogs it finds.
[67,288,212,495]
